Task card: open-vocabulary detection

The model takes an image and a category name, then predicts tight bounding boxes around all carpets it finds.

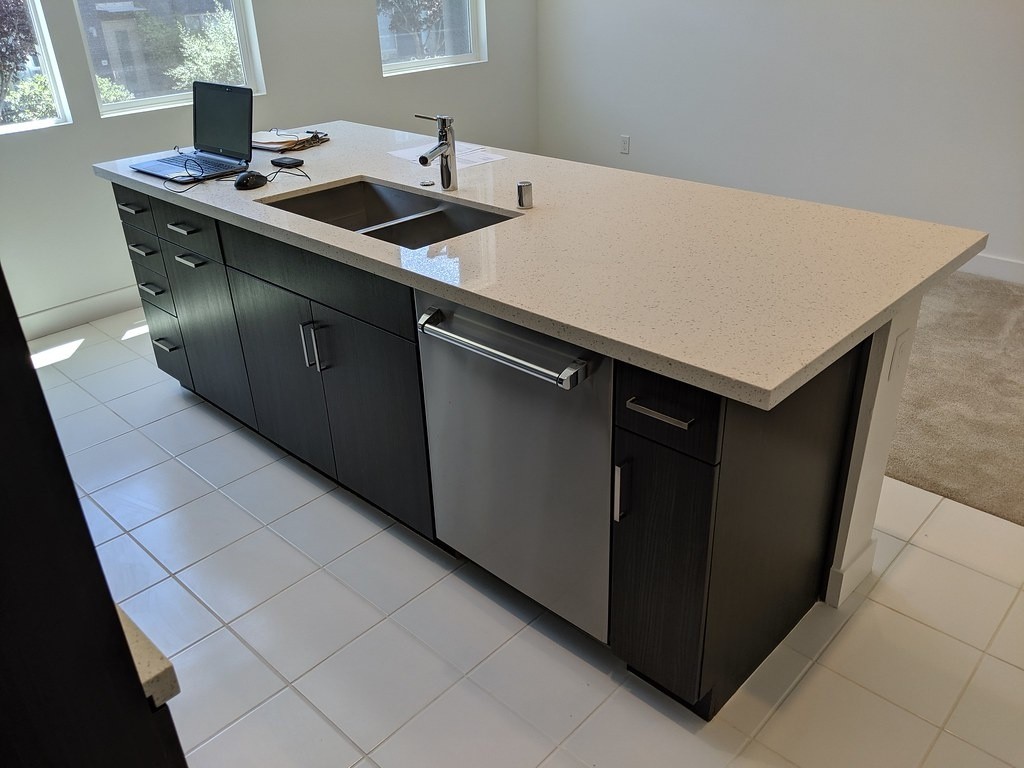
[882,269,1024,526]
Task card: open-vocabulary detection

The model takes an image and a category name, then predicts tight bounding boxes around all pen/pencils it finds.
[304,130,325,134]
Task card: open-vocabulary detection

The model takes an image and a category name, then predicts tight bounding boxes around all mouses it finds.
[234,170,267,190]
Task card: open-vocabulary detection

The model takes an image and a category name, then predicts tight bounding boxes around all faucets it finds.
[412,113,458,191]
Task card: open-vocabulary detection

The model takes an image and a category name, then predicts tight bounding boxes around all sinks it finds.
[251,174,439,233]
[361,202,523,251]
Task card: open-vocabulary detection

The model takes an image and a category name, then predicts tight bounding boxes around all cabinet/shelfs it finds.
[217,218,435,541]
[111,181,194,391]
[147,195,258,432]
[607,334,874,722]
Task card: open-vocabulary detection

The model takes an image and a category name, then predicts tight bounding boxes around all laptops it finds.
[129,81,253,185]
